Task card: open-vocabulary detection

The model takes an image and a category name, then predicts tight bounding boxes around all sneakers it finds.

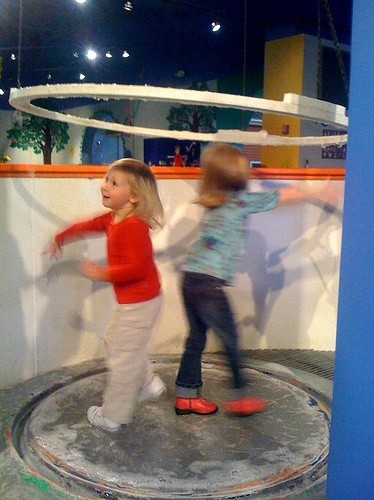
[138,376,166,402]
[87,406,120,432]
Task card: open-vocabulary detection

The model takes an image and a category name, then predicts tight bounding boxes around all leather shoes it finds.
[176,396,217,415]
[223,397,267,417]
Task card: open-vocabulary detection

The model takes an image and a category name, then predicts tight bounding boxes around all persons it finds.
[38,159,167,432]
[173,144,343,417]
[173,145,185,167]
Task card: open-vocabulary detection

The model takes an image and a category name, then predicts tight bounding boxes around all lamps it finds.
[123,0,133,10]
[211,17,221,32]
